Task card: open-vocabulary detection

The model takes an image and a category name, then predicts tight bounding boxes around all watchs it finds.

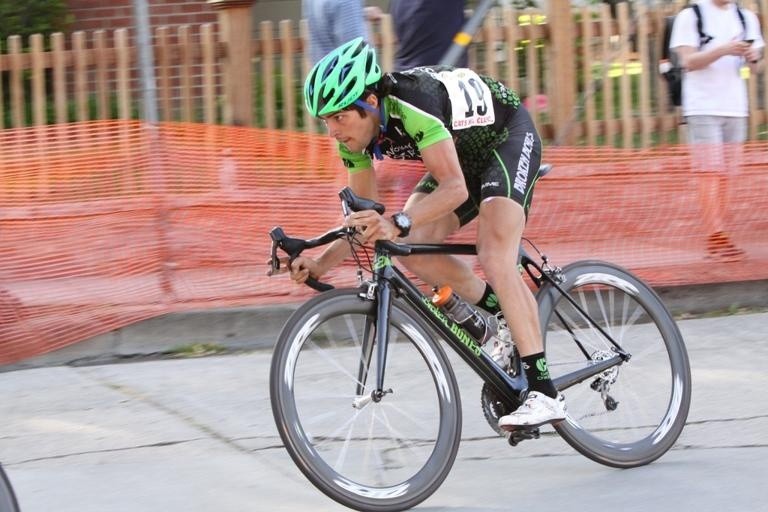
[389,212,412,239]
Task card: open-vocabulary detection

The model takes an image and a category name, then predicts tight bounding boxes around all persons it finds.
[264,36,567,428]
[388,0,470,72]
[302,0,384,67]
[670,1,768,262]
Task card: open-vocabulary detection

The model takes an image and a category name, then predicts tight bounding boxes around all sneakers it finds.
[477,311,518,375]
[496,388,567,430]
[701,227,750,264]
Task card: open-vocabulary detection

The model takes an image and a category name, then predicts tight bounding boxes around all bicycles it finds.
[268,161,692,512]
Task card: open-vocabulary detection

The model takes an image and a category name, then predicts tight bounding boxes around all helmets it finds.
[301,37,384,121]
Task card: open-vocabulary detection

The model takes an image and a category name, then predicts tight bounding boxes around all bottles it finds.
[658,58,675,74]
[432,286,492,345]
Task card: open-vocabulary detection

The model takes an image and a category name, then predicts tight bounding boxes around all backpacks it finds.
[657,3,713,108]
